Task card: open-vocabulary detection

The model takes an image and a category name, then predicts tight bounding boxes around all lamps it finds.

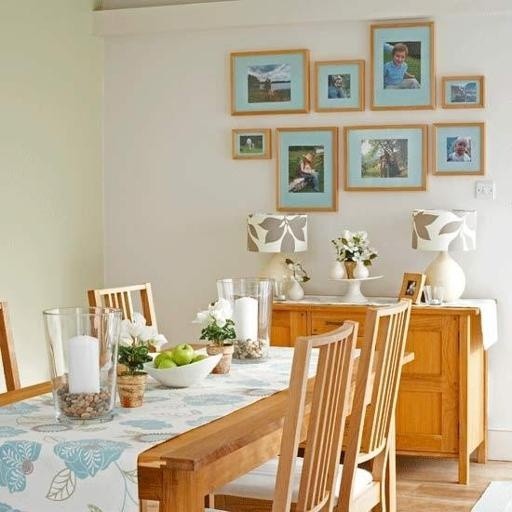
[247,213,308,279]
[409,209,478,301]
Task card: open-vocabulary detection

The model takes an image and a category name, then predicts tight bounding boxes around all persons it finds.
[383,44,421,89]
[448,139,470,161]
[297,152,322,191]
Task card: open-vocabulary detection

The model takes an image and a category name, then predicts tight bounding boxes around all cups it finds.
[423,285,447,305]
[271,279,287,300]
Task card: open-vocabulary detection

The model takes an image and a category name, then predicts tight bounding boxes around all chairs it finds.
[88,283,158,337]
[205,299,412,512]
[272,319,359,512]
[0,302,22,393]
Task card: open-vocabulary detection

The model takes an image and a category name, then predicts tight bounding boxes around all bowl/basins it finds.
[141,346,225,388]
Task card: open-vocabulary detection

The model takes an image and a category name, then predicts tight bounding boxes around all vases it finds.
[291,280,304,301]
[353,261,370,279]
[328,260,345,278]
[351,232,377,262]
[114,370,151,407]
[206,342,235,373]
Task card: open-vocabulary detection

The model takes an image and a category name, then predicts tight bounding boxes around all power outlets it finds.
[474,179,496,201]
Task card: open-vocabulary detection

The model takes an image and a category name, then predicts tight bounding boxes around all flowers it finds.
[284,258,309,281]
[195,300,241,345]
[329,234,348,261]
[116,313,158,375]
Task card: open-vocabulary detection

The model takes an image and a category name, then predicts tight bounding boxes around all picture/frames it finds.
[231,128,272,160]
[441,76,484,109]
[430,122,487,176]
[274,127,339,213]
[312,60,366,113]
[228,49,311,116]
[369,21,435,110]
[342,124,429,193]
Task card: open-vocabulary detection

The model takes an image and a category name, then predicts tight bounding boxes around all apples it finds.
[157,359,177,369]
[171,343,194,366]
[192,354,208,363]
[154,353,171,367]
